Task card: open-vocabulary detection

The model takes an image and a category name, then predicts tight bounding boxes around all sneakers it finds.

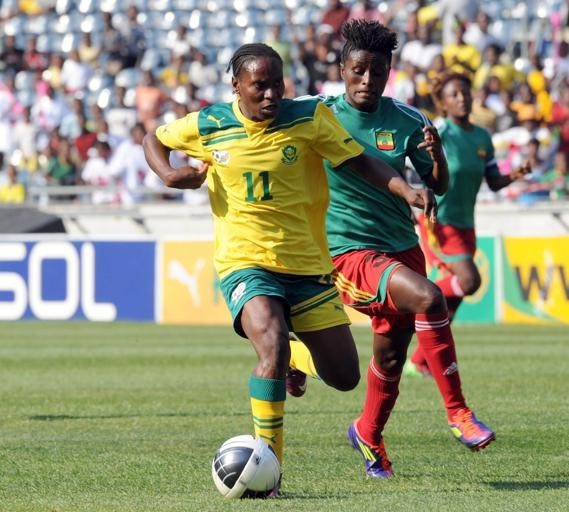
[447,409,495,451]
[347,417,396,481]
[286,336,308,396]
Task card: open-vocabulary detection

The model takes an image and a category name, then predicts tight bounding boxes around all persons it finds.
[143,44,437,496]
[315,21,496,481]
[0,0,569,206]
[406,74,534,379]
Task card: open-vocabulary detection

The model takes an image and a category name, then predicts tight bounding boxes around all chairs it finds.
[1,0,569,210]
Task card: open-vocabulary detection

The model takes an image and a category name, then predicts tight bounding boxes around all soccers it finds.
[211,435,281,500]
